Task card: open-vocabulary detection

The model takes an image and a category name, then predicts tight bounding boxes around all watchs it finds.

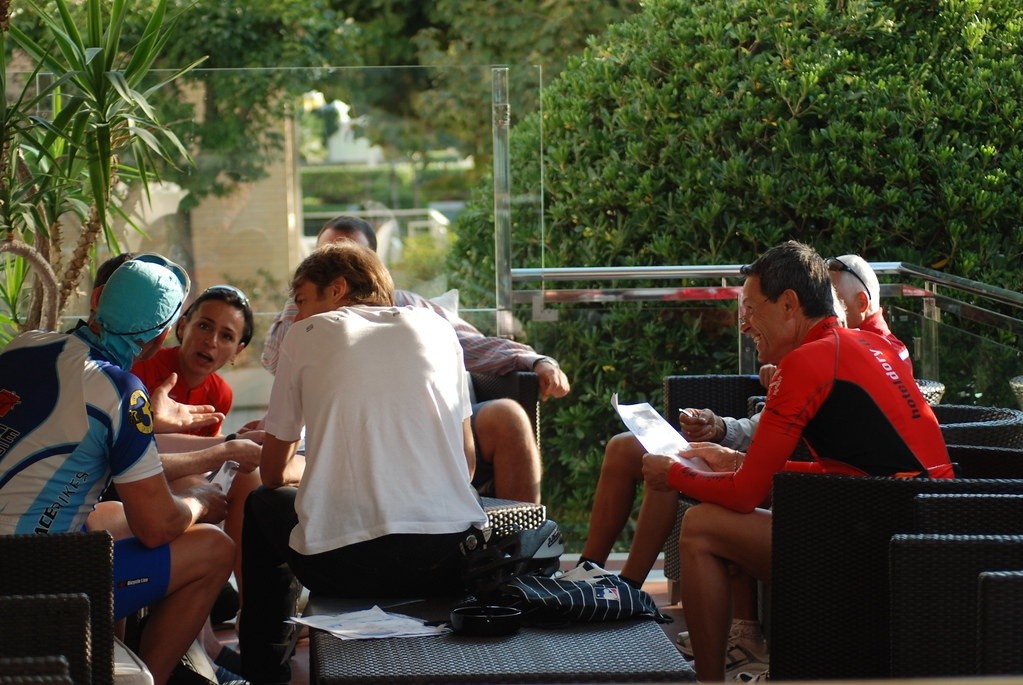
[225,433,238,442]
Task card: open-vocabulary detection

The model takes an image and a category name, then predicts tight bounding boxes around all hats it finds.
[93,258,185,344]
[175,285,253,347]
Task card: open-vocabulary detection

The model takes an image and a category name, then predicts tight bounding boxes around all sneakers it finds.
[674,619,764,661]
[734,669,769,683]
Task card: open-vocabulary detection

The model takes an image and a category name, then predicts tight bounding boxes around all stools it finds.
[482,497,547,540]
[113,635,156,685]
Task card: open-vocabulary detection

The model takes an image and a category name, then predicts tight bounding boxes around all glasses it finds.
[825,256,872,300]
[98,253,191,335]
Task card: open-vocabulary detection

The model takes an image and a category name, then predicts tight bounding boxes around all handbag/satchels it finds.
[498,559,667,626]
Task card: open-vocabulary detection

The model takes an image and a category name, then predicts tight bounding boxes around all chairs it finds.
[662,376,946,606]
[767,443,1023,682]
[470,370,541,502]
[890,532,1023,679]
[931,404,1023,449]
[916,492,1023,535]
[0,530,114,685]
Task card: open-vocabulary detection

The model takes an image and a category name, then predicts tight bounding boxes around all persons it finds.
[260,217,569,504]
[0,251,266,684]
[643,241,953,685]
[236,241,490,685]
[550,255,912,684]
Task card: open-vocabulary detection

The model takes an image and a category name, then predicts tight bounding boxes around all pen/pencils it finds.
[679,409,693,418]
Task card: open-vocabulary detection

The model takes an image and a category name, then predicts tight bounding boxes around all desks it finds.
[308,588,697,685]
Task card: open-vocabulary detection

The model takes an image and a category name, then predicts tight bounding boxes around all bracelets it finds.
[734,450,738,472]
